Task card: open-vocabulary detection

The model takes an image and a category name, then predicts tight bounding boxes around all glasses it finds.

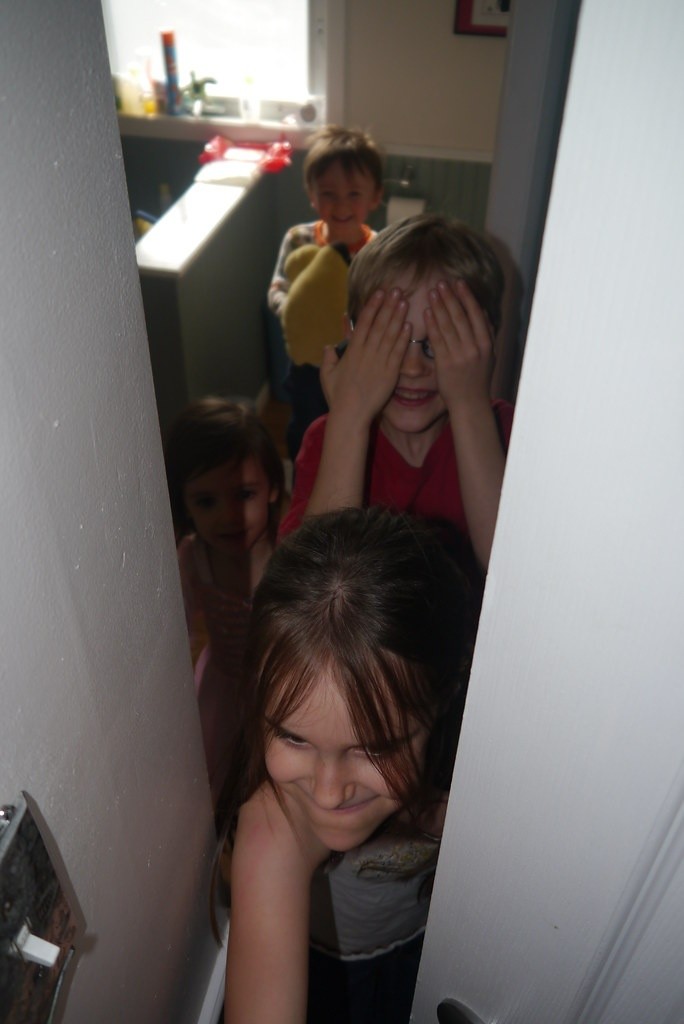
[408,334,438,359]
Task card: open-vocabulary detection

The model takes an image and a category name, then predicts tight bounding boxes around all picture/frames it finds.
[454,0,513,36]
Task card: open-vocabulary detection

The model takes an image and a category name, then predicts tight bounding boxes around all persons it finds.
[267,130,382,494]
[275,215,514,575]
[207,503,486,1024]
[162,394,284,815]
